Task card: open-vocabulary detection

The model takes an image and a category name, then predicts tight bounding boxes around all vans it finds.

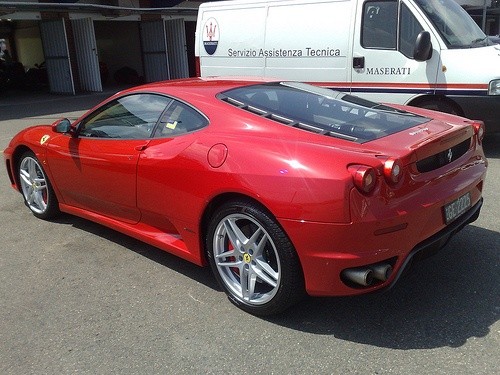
[195,0,499,139]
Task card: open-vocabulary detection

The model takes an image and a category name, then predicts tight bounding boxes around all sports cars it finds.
[2,78,488,317]
